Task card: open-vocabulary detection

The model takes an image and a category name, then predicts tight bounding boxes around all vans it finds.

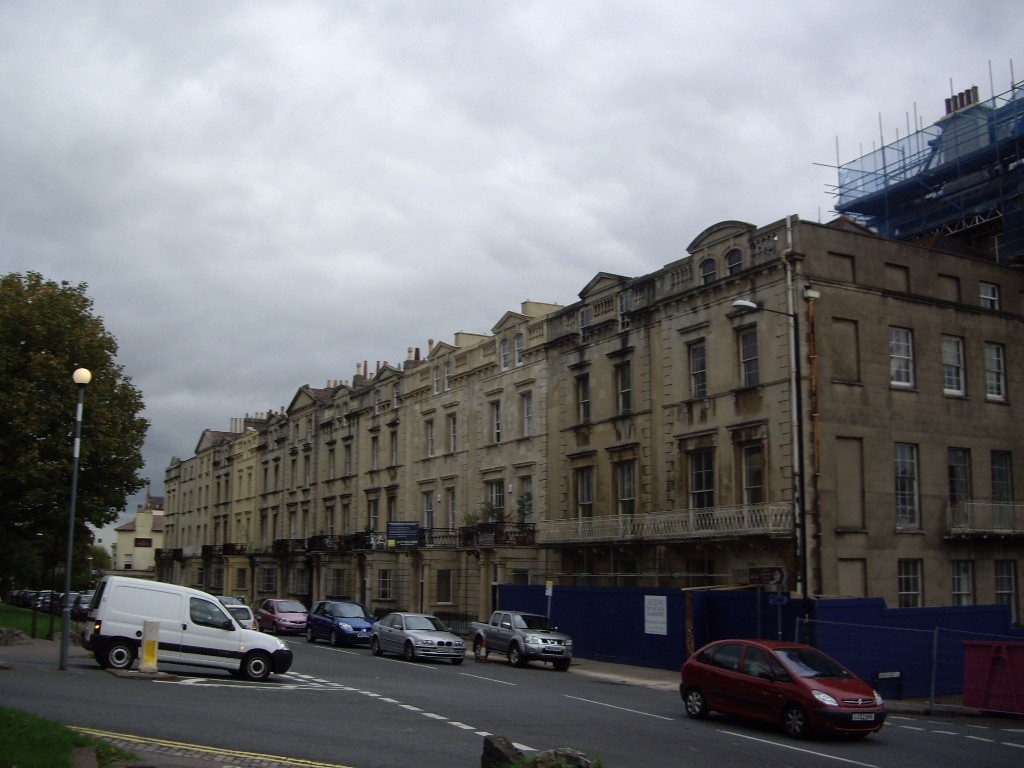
[81,575,294,682]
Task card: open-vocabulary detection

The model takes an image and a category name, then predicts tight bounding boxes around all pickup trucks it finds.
[470,610,574,671]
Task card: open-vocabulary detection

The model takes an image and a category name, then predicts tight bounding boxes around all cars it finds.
[256,599,309,636]
[370,612,467,664]
[305,600,378,648]
[676,639,888,739]
[6,590,96,622]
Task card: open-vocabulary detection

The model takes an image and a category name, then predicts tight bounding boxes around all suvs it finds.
[190,596,259,632]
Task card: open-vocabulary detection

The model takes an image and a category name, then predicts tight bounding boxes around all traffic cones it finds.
[478,636,489,663]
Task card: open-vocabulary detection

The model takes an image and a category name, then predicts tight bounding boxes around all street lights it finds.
[59,367,92,670]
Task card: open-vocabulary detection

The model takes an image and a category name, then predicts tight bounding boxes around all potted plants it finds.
[461,493,532,546]
